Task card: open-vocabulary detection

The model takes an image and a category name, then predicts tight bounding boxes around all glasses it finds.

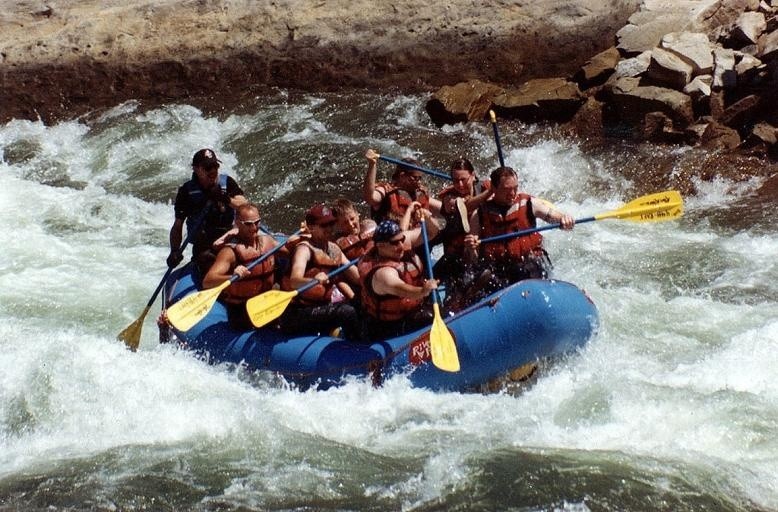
[454,176,475,185]
[405,170,422,182]
[200,162,221,171]
[238,219,263,227]
[389,235,407,246]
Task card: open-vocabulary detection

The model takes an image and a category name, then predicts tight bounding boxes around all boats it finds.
[157,231,599,396]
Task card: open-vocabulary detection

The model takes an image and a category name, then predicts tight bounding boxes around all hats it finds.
[304,207,337,224]
[193,148,223,163]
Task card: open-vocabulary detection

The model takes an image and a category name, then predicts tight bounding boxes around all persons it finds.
[167,149,576,340]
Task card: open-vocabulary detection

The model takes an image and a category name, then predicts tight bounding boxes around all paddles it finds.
[420,218,461,373]
[246,257,361,329]
[167,219,310,332]
[117,203,212,351]
[480,190,683,244]
